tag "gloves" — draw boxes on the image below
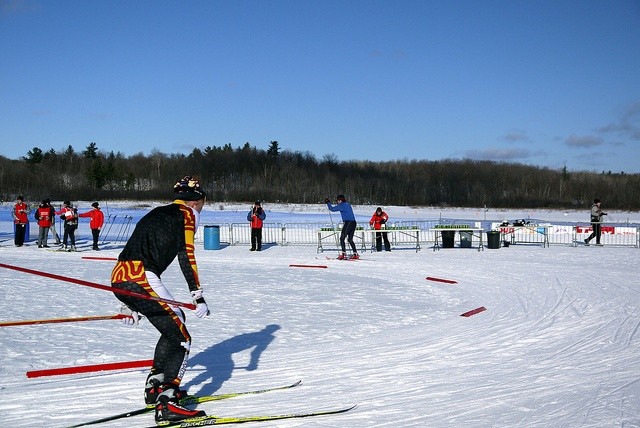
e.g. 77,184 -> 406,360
190,290 -> 208,319
120,303 -> 139,326
325,198 -> 330,203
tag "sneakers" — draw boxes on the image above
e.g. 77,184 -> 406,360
338,255 -> 347,260
70,245 -> 76,250
59,244 -> 66,250
155,383 -> 206,426
350,254 -> 359,259
144,369 -> 187,409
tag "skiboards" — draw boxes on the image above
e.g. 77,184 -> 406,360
46,249 -> 84,252
56,380 -> 356,427
317,256 -> 368,261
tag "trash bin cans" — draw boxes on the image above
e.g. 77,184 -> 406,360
487,231 -> 500,248
14,220 -> 30,242
441,231 -> 455,248
204,225 -> 220,250
459,231 -> 473,248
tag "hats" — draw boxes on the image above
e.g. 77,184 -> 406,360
172,175 -> 206,201
594,199 -> 600,204
91,202 -> 99,209
337,194 -> 344,198
17,196 -> 23,201
255,202 -> 261,205
64,201 -> 70,205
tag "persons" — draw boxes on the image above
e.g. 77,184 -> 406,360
585,200 -> 607,247
11,195 -> 31,248
369,207 -> 392,252
247,199 -> 266,251
325,193 -> 360,260
109,174 -> 208,425
79,202 -> 105,251
34,198 -> 55,249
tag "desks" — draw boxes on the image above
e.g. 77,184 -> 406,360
497,223 -> 552,248
366,229 -> 421,253
429,228 -> 484,251
317,230 -> 366,253
